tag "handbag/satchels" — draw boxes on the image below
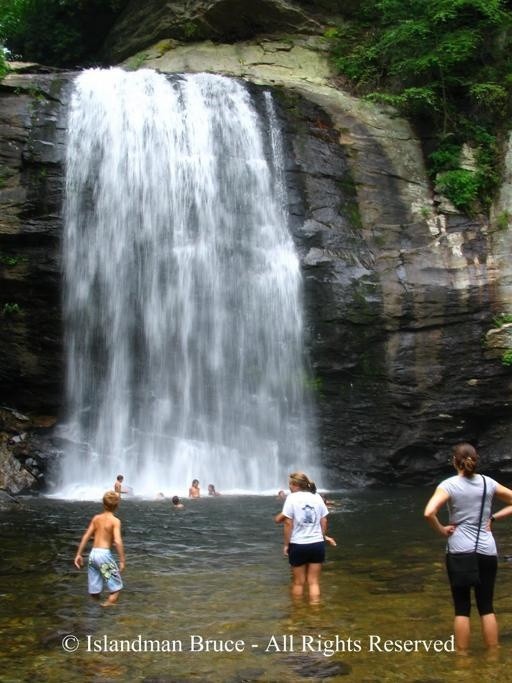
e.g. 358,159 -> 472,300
445,554 -> 476,574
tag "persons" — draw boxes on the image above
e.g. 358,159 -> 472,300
73,490 -> 126,604
274,472 -> 337,596
114,474 -> 128,499
423,442 -> 512,653
207,483 -> 221,497
171,495 -> 184,508
155,492 -> 164,500
188,479 -> 201,498
291,596 -> 320,604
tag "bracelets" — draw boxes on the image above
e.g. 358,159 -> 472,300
489,515 -> 495,522
